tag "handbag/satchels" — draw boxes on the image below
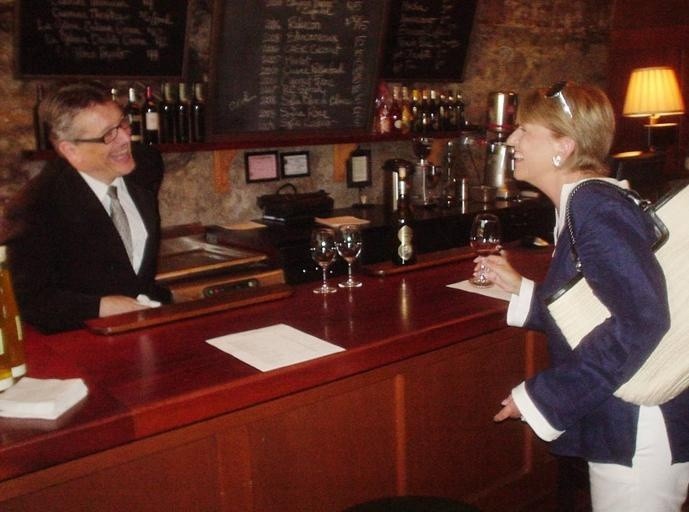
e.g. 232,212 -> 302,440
544,178 -> 689,407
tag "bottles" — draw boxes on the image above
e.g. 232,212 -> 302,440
420,86 -> 430,131
392,176 -> 417,266
410,88 -> 422,133
176,82 -> 190,143
375,95 -> 390,134
438,93 -> 448,131
429,88 -> 438,132
32,84 -> 52,150
159,81 -> 175,142
124,87 -> 143,144
190,81 -> 205,143
401,86 -> 410,132
142,86 -> 159,144
455,89 -> 465,130
389,86 -> 401,133
447,89 -> 457,131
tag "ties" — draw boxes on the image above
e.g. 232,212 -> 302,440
107,186 -> 134,269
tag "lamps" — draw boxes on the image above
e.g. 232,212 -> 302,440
622,67 -> 685,123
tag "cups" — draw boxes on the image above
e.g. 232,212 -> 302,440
337,224 -> 363,288
472,213 -> 502,286
311,228 -> 337,294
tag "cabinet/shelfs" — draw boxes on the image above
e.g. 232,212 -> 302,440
22,123 -> 487,163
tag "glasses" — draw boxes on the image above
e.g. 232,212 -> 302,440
56,113 -> 131,145
542,78 -> 573,119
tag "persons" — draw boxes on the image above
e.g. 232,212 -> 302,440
6,83 -> 173,334
472,80 -> 689,512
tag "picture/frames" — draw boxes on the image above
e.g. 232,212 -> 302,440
345,147 -> 373,188
280,152 -> 311,179
244,151 -> 280,184
